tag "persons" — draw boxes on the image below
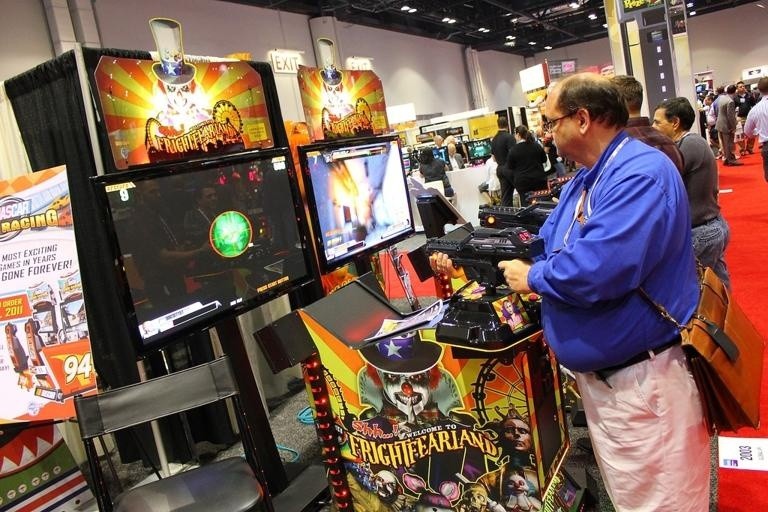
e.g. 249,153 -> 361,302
502,297 -> 527,331
506,125 -> 546,207
696,76 -> 768,182
428,71 -> 711,512
609,75 -> 684,176
650,97 -> 730,295
491,116 -> 529,208
187,183 -> 270,285
419,134 -> 464,206
476,417 -> 539,498
127,181 -> 238,307
529,125 -> 579,180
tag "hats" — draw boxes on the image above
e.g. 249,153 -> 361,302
359,329 -> 444,377
315,37 -> 343,85
145,16 -> 197,88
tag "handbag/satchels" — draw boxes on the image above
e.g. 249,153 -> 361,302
681,265 -> 768,433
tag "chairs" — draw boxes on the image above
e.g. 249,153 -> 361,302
73,354 -> 276,512
424,180 -> 457,208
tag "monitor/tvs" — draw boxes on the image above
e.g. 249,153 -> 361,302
401,154 -> 411,169
89,147 -> 320,351
465,139 -> 493,161
297,133 -> 416,274
432,146 -> 449,165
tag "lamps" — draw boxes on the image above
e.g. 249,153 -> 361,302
685,1 -> 697,16
401,5 -> 554,50
568,0 -> 608,29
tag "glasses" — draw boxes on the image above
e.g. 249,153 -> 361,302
543,112 -> 577,133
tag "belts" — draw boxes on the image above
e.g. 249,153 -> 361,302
595,336 -> 680,376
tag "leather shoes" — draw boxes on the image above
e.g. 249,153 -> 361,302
729,162 -> 743,165
